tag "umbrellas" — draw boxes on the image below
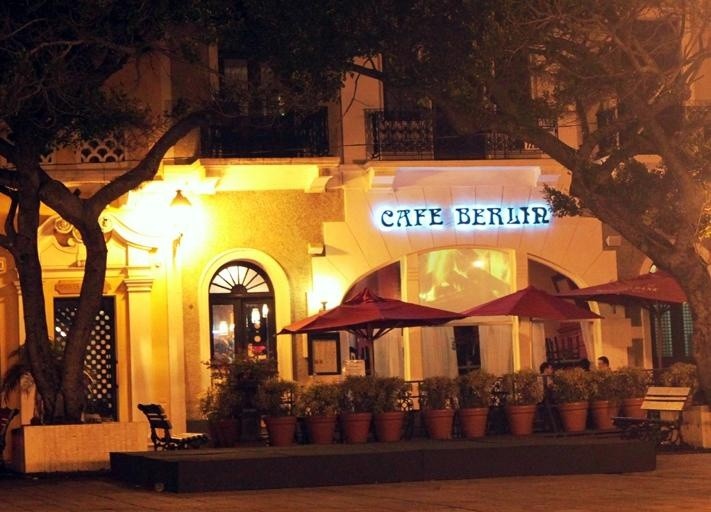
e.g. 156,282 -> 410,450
554,270 -> 689,369
273,286 -> 471,377
460,282 -> 606,369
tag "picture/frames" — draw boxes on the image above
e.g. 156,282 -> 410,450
307,333 -> 341,374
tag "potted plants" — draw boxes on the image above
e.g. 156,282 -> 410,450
201,348 -> 277,447
271,365 -> 646,445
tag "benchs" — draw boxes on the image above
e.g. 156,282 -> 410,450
611,387 -> 691,450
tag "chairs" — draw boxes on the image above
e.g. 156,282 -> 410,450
137,404 -> 209,451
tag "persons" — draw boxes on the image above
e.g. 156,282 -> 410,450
599,356 -> 613,374
539,363 -> 553,375
579,358 -> 592,372
